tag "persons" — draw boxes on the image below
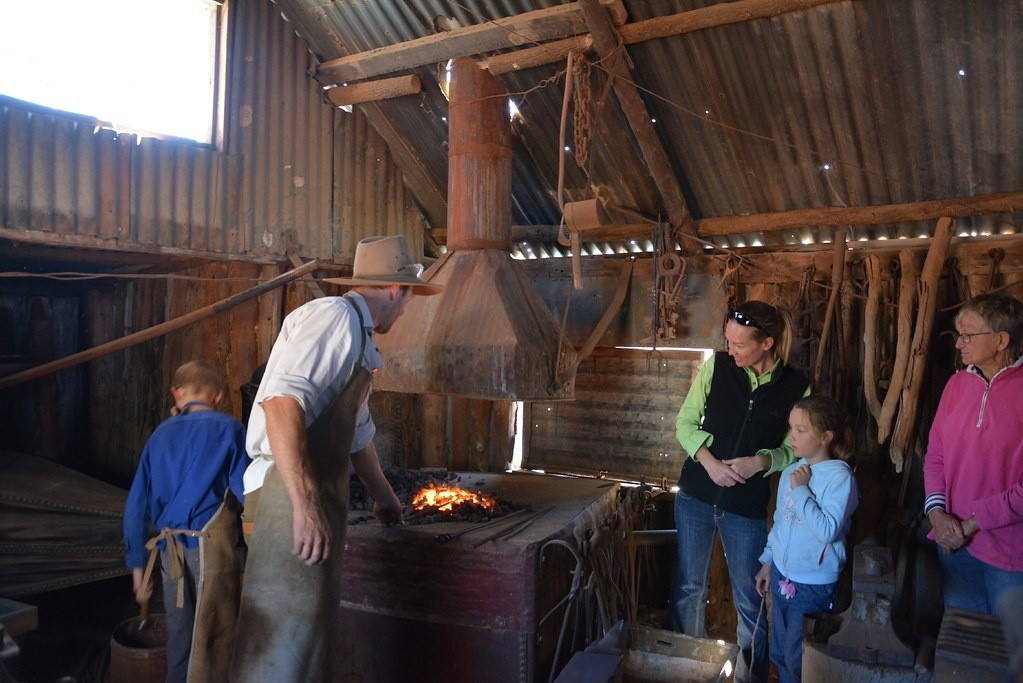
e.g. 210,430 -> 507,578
922,291 -> 1023,682
227,234 -> 451,683
120,359 -> 249,683
674,300 -> 813,683
754,394 -> 862,683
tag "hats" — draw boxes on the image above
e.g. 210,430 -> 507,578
323,234 -> 445,296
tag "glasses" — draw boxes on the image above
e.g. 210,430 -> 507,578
951,329 -> 993,344
727,310 -> 770,337
